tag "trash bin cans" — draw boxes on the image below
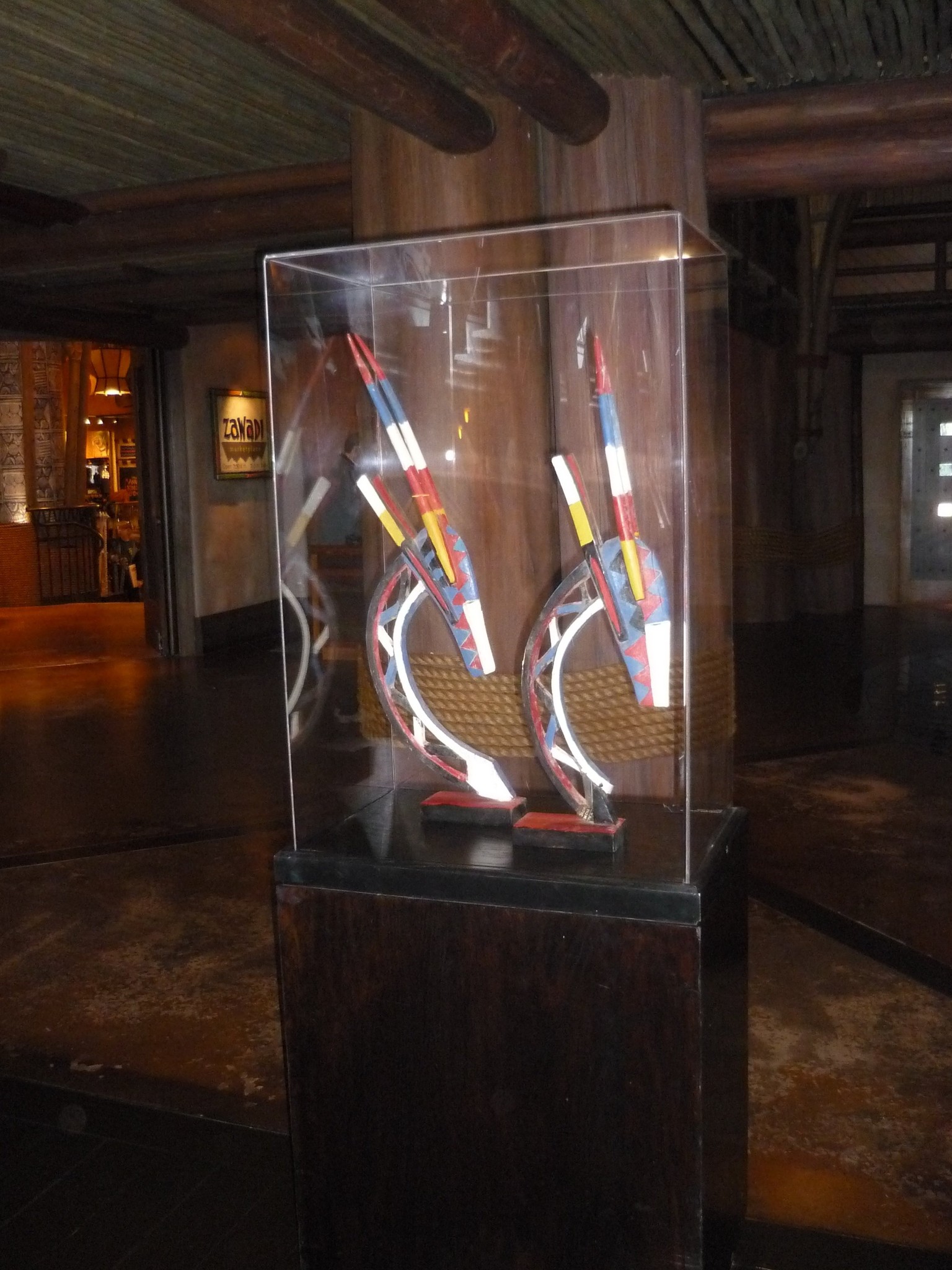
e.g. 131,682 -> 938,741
26,502 -> 110,604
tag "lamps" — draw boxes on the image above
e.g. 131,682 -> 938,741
96,417 -> 103,425
90,342 -> 131,396
